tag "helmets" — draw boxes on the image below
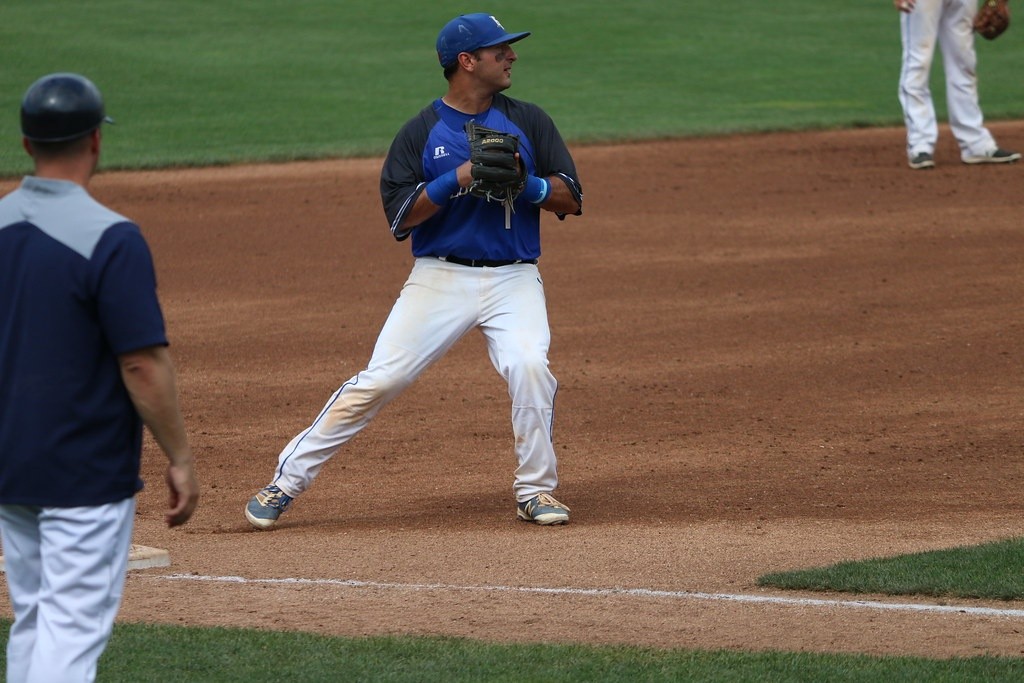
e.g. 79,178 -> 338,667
21,73 -> 115,141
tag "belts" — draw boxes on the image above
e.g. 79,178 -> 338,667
426,252 -> 536,267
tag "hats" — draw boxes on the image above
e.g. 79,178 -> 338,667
436,13 -> 531,68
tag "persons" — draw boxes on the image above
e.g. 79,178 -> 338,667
894,0 -> 1022,170
245,13 -> 584,532
0,73 -> 200,683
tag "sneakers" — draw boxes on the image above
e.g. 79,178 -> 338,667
516,493 -> 571,525
961,148 -> 1022,164
245,481 -> 294,531
908,152 -> 934,168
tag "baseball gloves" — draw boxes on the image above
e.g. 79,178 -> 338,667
461,118 -> 530,199
973,0 -> 1010,41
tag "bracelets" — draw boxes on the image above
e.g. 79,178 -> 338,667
425,168 -> 461,207
517,174 -> 551,208
